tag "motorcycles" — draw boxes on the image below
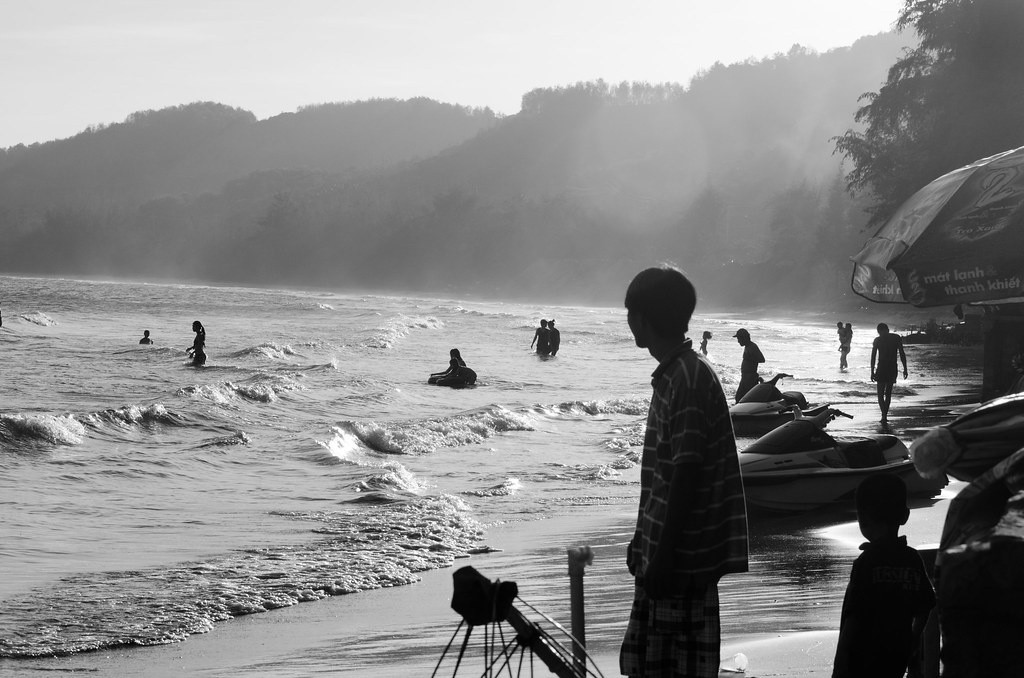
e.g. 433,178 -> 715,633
731,373 -> 811,428
740,402 -> 921,511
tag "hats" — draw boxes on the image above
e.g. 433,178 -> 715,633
732,328 -> 749,338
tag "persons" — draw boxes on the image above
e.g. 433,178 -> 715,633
832,474 -> 938,678
430,349 -> 477,385
620,267 -> 749,678
186,321 -> 206,364
870,323 -> 909,422
531,319 -> 560,356
837,321 -> 853,368
732,328 -> 765,403
140,330 -> 153,344
700,331 -> 712,356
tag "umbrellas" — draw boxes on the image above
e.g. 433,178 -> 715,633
849,146 -> 1024,308
